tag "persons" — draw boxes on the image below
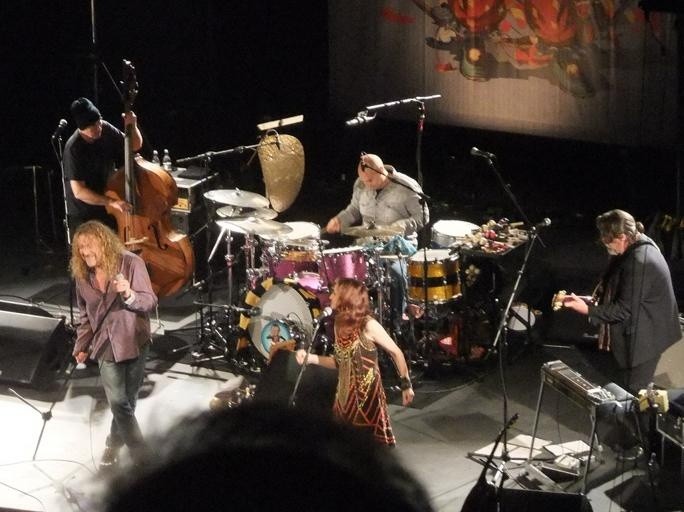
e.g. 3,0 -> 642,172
563,208 -> 683,463
264,323 -> 286,343
295,277 -> 416,449
326,154 -> 430,337
60,97 -> 144,246
66,218 -> 160,479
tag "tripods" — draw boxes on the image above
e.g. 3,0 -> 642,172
57,139 -> 84,329
408,199 -> 463,395
167,200 -> 287,376
366,251 -> 408,393
9,293 -> 119,460
504,239 -> 577,367
467,235 -> 555,484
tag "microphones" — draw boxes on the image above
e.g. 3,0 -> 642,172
471,147 -> 496,159
360,152 -> 366,172
275,136 -> 282,151
529,217 -> 552,232
117,273 -> 125,306
52,118 -> 67,139
310,307 -> 332,323
344,111 -> 377,126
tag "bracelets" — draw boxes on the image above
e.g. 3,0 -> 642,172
400,375 -> 411,390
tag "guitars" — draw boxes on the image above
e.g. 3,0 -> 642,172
551,281 -> 605,327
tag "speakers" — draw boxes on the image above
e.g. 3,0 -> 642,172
461,485 -> 594,512
0,310 -> 65,392
0,298 -> 53,318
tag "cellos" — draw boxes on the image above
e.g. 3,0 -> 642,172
104,58 -> 196,299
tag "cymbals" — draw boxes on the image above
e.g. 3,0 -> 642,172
203,190 -> 270,208
215,206 -> 293,236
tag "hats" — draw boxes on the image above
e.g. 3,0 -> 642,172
69,97 -> 102,131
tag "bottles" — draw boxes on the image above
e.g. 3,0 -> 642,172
162,148 -> 171,173
151,150 -> 160,166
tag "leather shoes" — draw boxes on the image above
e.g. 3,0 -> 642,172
620,444 -> 653,460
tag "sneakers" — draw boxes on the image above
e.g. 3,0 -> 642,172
99,437 -> 119,471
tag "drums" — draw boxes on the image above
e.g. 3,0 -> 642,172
406,248 -> 463,306
246,270 -> 337,361
315,246 -> 369,294
260,221 -> 330,284
428,219 -> 481,249
